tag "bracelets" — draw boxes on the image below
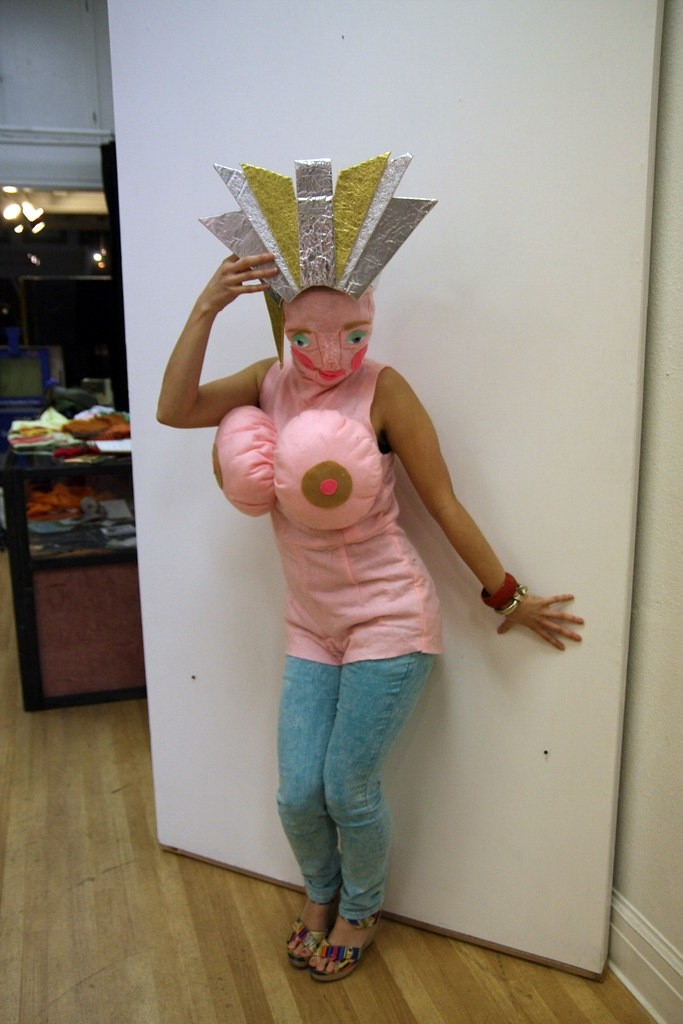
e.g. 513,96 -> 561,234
480,573 -> 527,614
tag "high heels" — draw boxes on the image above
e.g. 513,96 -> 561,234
308,909 -> 381,981
287,896 -> 339,968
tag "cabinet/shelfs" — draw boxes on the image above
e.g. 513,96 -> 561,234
0,448 -> 146,714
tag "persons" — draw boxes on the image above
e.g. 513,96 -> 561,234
157,153 -> 584,982
